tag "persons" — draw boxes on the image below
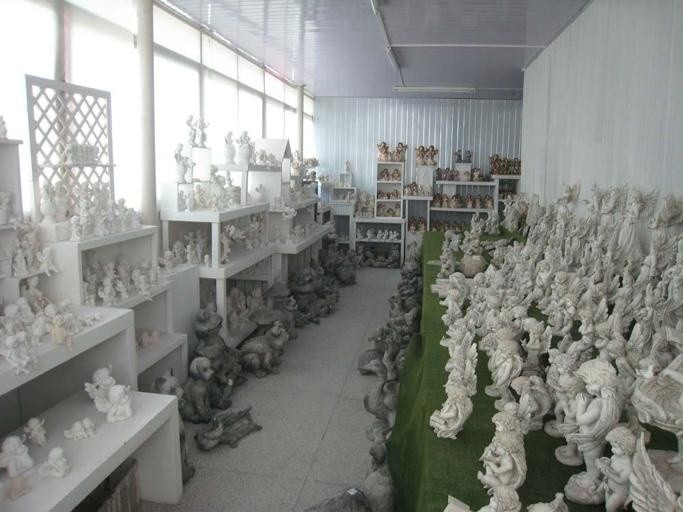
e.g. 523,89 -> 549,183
0,115 -> 683,512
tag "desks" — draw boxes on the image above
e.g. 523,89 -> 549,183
388,227 -> 678,512
